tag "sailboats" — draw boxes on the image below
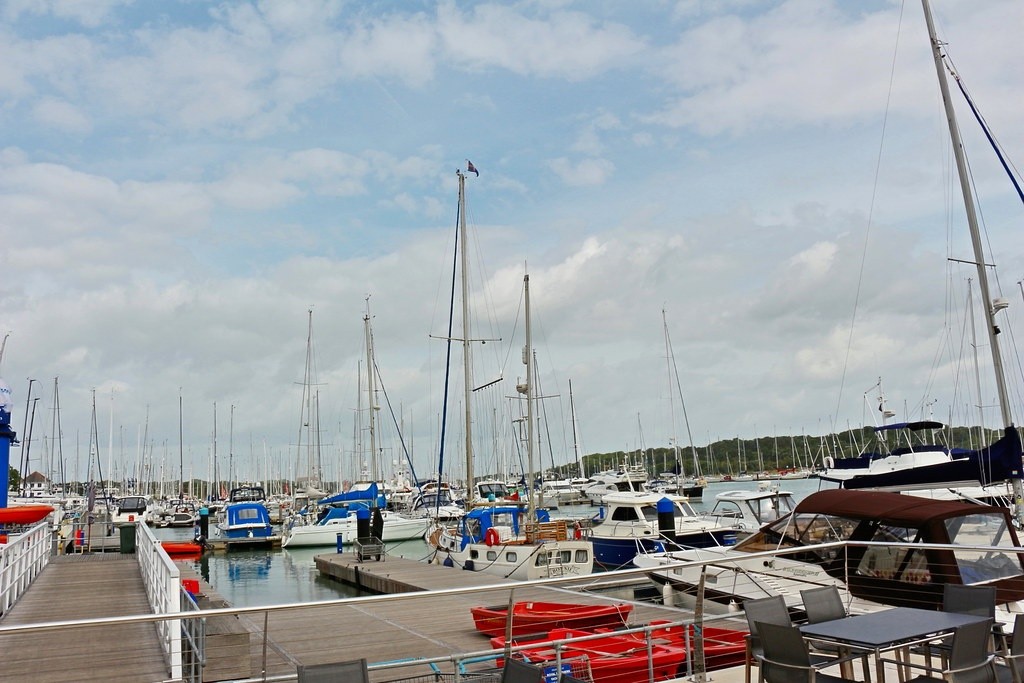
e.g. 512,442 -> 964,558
1,0 -> 1023,639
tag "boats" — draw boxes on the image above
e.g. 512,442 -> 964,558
488,626 -> 689,683
592,618 -> 757,669
470,600 -> 635,639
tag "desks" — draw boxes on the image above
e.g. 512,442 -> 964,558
800,605 -> 991,683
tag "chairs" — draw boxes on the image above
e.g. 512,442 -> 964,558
743,584 -> 1024,683
